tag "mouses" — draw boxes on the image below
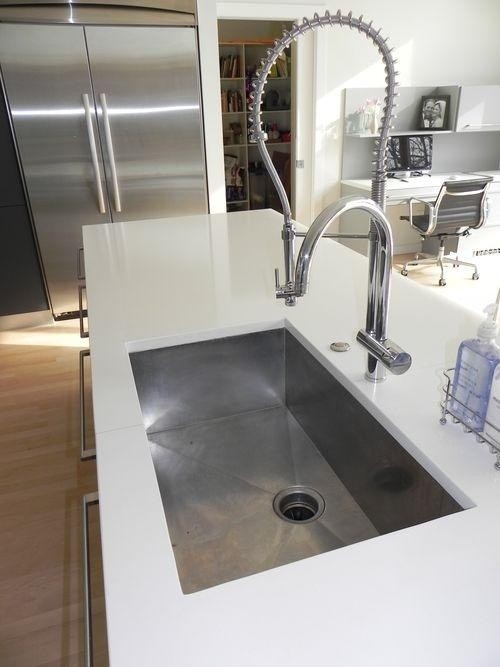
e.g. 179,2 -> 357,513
449,174 -> 460,181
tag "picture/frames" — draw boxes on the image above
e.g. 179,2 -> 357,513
417,93 -> 451,129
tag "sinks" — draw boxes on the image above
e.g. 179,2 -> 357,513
122,318 -> 476,594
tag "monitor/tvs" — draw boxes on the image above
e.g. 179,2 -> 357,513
384,134 -> 434,182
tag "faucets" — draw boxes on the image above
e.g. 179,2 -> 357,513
294,194 -> 413,383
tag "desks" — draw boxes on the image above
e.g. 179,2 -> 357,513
336,167 -> 500,255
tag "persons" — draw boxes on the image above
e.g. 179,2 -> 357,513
429,100 -> 446,128
422,98 -> 436,128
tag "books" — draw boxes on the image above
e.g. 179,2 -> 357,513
218,50 -> 291,213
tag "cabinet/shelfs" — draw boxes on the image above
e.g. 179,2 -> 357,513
218,39 -> 294,216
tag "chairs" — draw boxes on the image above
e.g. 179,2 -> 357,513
398,176 -> 492,286
